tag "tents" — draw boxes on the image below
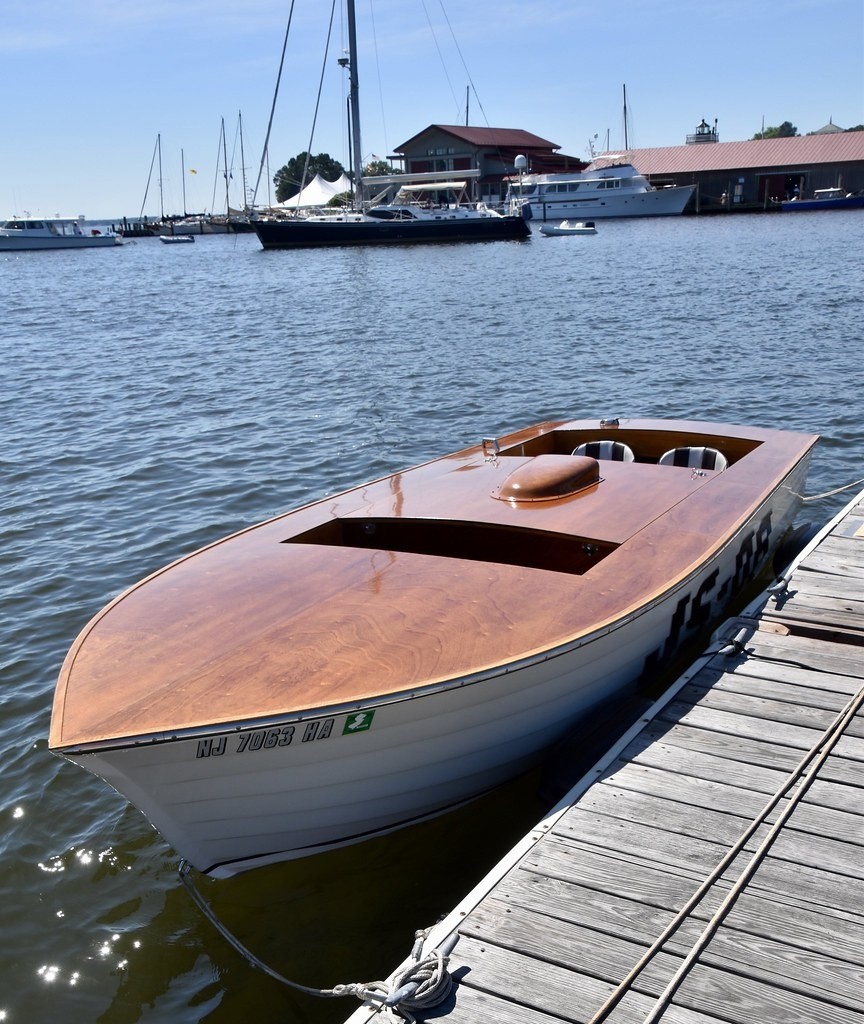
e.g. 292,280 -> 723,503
270,171 -> 358,219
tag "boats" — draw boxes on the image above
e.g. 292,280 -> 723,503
502,154 -> 698,217
159,234 -> 195,244
44,411 -> 823,882
781,187 -> 864,212
0,219 -> 125,252
540,221 -> 598,236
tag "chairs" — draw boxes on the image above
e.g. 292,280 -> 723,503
571,440 -> 635,462
657,446 -> 728,471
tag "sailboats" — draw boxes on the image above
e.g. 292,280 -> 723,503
110,110 -> 271,239
246,0 -> 537,249
